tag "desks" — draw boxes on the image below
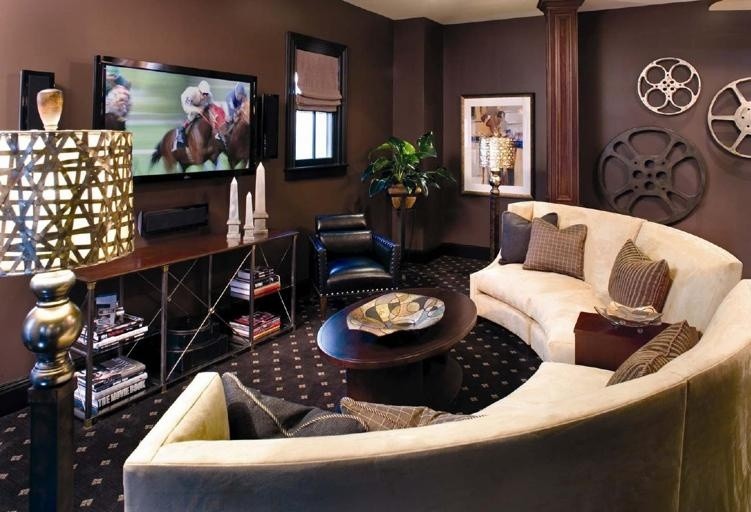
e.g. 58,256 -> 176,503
71,229 -> 299,428
574,312 -> 673,372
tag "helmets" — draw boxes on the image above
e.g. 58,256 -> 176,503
235,84 -> 246,97
198,80 -> 210,93
108,68 -> 119,75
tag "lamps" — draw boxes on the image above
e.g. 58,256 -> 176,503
479,136 -> 515,263
0,88 -> 136,511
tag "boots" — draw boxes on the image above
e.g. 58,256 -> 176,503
177,120 -> 189,144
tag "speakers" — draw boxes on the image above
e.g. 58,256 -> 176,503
259,93 -> 280,160
18,68 -> 55,130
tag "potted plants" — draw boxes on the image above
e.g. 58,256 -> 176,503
361,129 -> 456,210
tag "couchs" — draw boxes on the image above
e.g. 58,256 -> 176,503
469,199 -> 744,363
306,212 -> 401,325
122,278 -> 751,512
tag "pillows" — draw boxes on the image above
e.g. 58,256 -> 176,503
523,217 -> 588,281
221,371 -> 368,442
337,396 -> 486,430
608,238 -> 671,312
498,211 -> 559,265
606,319 -> 699,386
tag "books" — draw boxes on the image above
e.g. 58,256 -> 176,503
229,266 -> 281,296
73,355 -> 149,415
229,310 -> 281,340
76,294 -> 149,351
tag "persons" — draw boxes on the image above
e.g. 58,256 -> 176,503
106,66 -> 132,95
225,82 -> 247,122
176,79 -> 214,145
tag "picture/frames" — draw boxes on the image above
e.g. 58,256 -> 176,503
459,92 -> 535,199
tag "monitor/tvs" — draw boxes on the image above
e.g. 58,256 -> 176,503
92,54 -> 259,190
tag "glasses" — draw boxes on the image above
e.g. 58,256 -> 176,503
199,89 -> 209,96
110,74 -> 117,78
